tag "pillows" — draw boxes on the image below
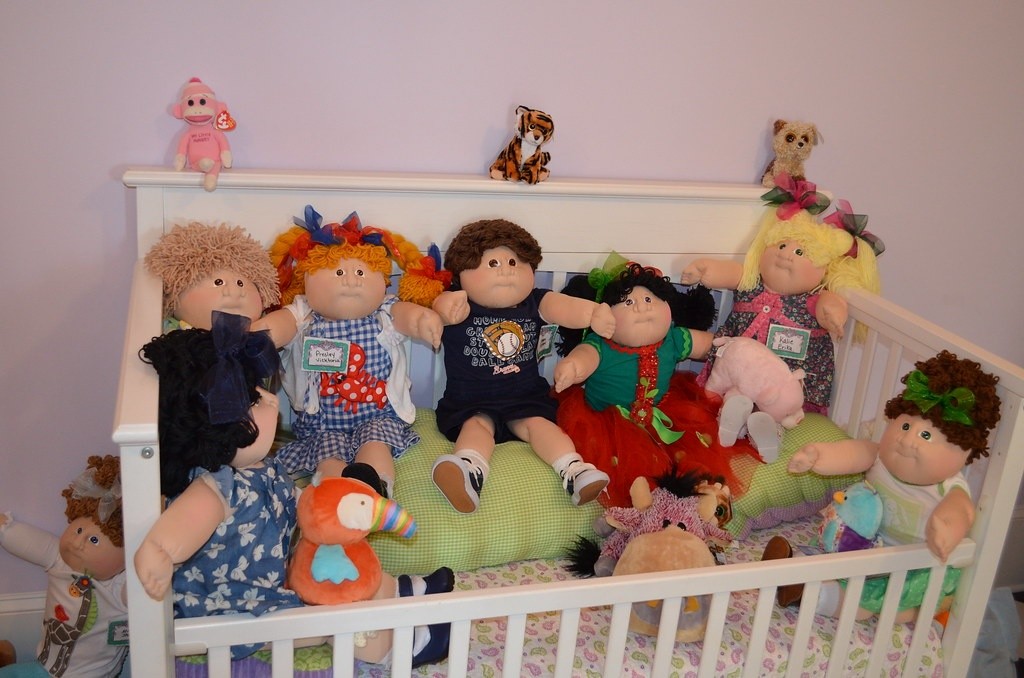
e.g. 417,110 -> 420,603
368,409 -> 608,577
722,414 -> 865,541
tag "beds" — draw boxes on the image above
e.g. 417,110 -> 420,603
112,166 -> 1024,678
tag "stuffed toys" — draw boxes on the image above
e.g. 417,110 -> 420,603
0,77 -> 1003,678
432,219 -> 616,514
245,205 -> 453,499
